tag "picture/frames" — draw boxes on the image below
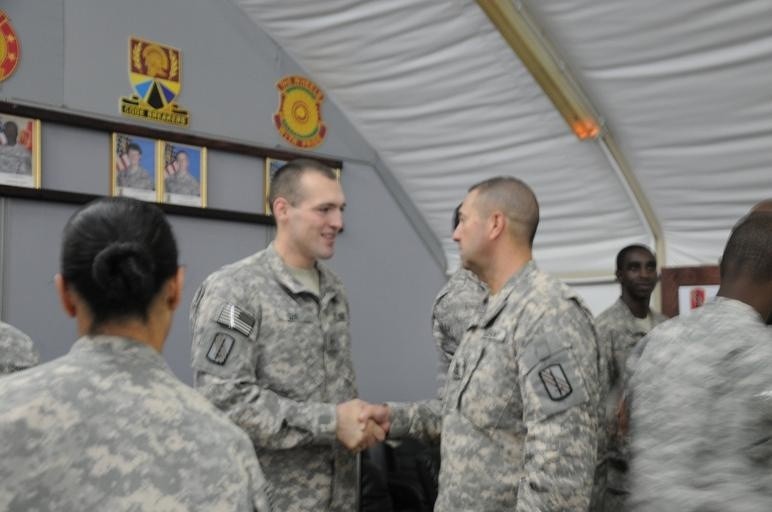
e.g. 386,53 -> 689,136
110,130 -> 161,204
0,112 -> 43,191
158,138 -> 209,211
266,155 -> 289,218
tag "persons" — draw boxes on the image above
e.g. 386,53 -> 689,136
621,196 -> 772,512
193,155 -> 385,511
359,175 -> 607,512
117,143 -> 153,190
0,197 -> 268,510
167,151 -> 200,197
0,123 -> 32,175
429,201 -> 493,393
592,244 -> 670,510
